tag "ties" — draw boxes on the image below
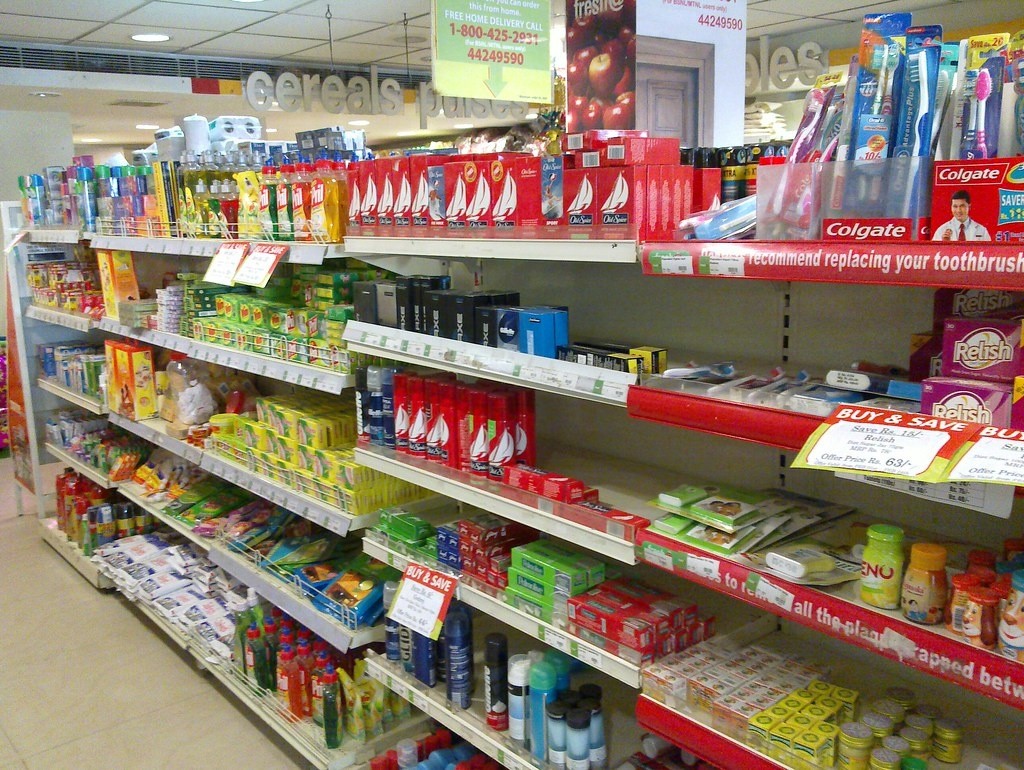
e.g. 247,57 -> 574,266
959,224 -> 966,241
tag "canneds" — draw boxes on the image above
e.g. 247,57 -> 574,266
837,686 -> 962,770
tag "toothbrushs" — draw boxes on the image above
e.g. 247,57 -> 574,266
678,11 -> 1024,242
649,360 -> 823,406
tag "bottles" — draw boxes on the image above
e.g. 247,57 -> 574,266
261,160 -> 350,243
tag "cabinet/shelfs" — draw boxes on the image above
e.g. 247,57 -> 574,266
343,224 -> 1024,770
1,199 -> 163,592
90,216 -> 461,770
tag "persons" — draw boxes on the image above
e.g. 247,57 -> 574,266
696,501 -> 742,547
932,191 -> 992,241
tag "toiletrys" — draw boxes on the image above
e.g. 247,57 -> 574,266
353,276 -> 569,361
355,364 -> 537,480
383,582 -> 606,770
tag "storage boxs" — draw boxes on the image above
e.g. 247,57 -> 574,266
348,129 -> 721,243
35,339 -> 106,400
814,287 -> 1023,435
372,503 -> 859,769
95,248 -> 159,329
215,393 -> 441,515
238,126 -> 368,164
46,411 -> 151,481
26,259 -> 105,313
103,339 -> 157,421
95,162 -> 184,236
177,257 -> 409,373
354,270 -> 667,383
756,156 -> 1024,242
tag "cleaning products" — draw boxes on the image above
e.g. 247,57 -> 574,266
17,153 -> 174,236
232,586 -> 424,750
55,466 -> 163,556
176,152 -> 349,243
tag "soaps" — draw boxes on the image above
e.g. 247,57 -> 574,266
215,389 -> 435,515
176,257 -> 396,374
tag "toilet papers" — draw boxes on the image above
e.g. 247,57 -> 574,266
102,151 -> 129,166
130,116 -> 298,167
